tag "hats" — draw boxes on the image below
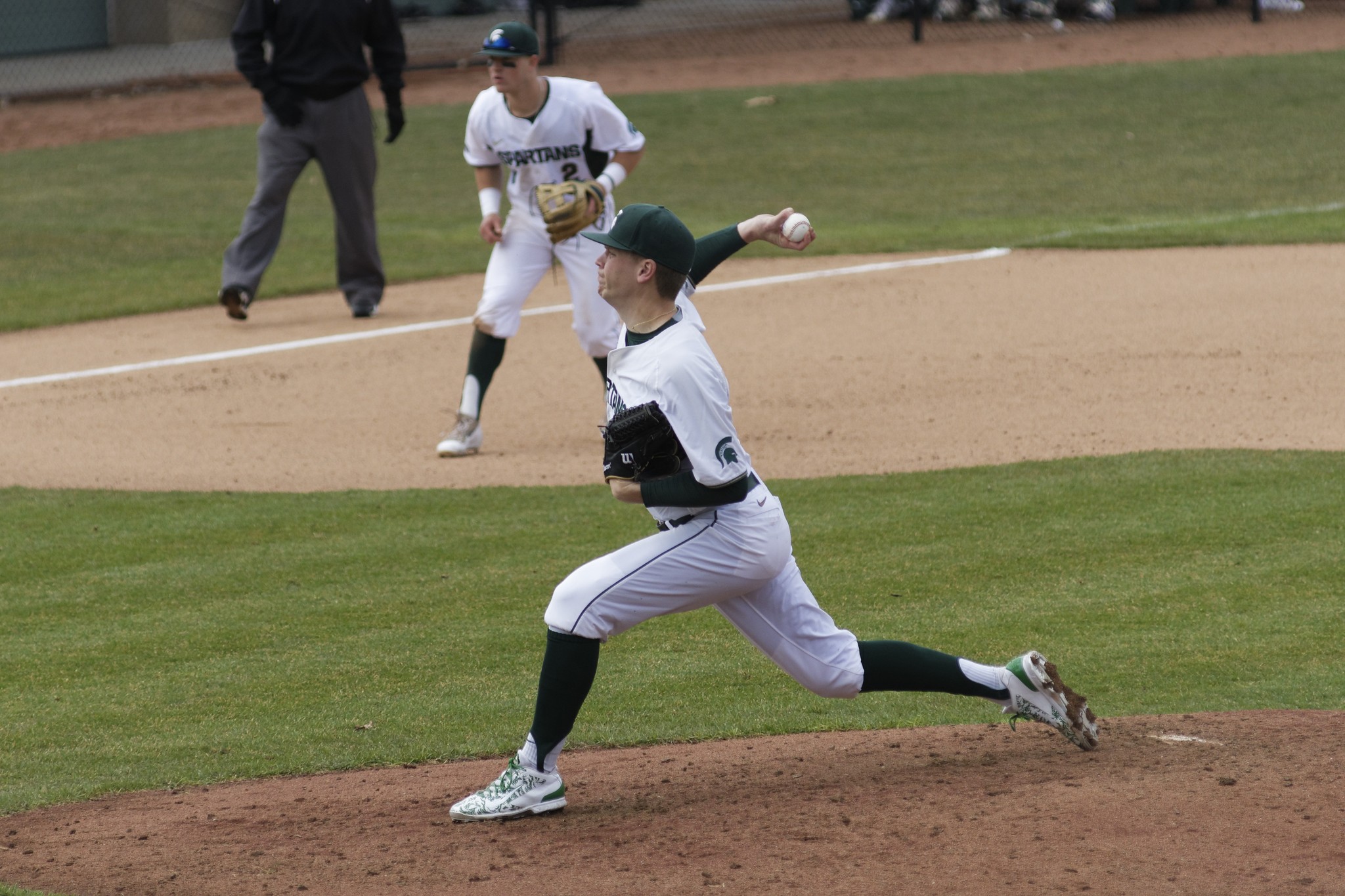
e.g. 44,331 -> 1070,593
580,202 -> 698,274
474,21 -> 540,59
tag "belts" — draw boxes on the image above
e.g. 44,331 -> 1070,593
656,472 -> 758,531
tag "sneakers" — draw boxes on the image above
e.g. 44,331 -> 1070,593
434,411 -> 481,456
998,649 -> 1098,751
449,748 -> 569,822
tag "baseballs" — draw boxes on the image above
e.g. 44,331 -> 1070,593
782,211 -> 812,244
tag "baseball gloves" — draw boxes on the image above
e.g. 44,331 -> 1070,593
602,402 -> 681,482
536,179 -> 606,245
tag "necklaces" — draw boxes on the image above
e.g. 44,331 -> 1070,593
626,309 -> 672,328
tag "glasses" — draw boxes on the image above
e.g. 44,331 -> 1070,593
483,37 -> 534,55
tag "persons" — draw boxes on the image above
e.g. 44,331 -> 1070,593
860,0 -> 1120,33
217,0 -> 411,320
437,18 -> 646,459
526,0 -> 558,66
447,205 -> 1101,827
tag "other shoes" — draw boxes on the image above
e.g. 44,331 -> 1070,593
352,302 -> 372,317
218,284 -> 247,321
